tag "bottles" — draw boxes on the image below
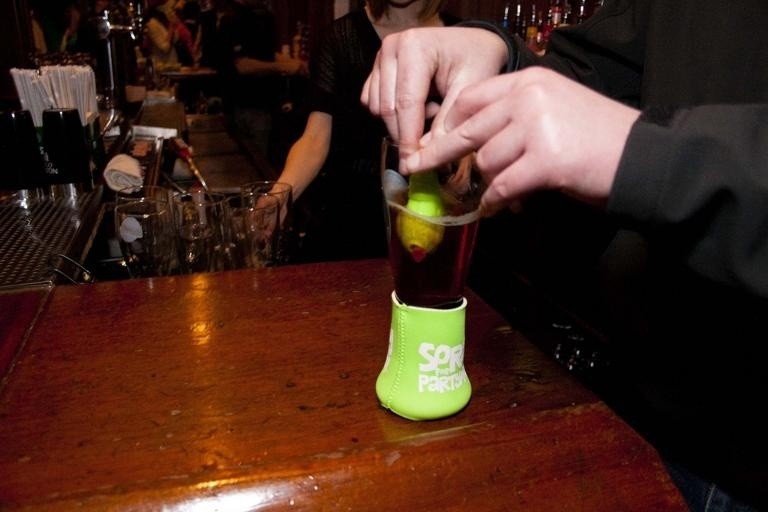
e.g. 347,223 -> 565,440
501,2 -> 591,55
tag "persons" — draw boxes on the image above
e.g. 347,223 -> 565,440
360,0 -> 767,300
250,0 -> 465,263
146,0 -> 311,114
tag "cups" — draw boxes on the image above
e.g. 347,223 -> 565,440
114,181 -> 294,276
375,132 -> 475,421
